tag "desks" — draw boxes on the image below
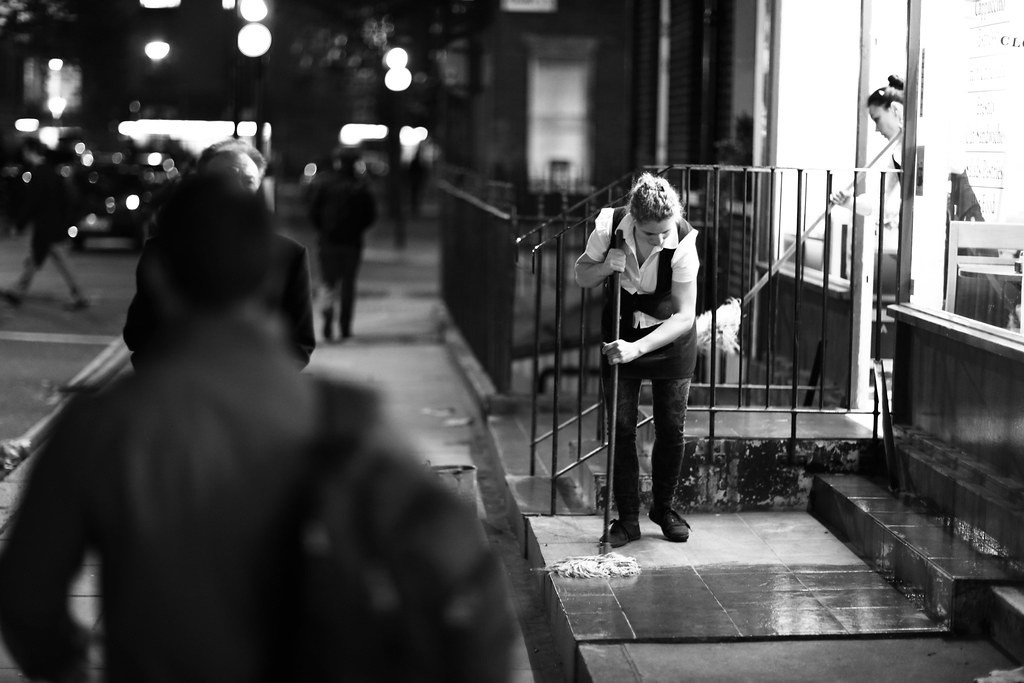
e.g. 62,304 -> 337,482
959,263 -> 1023,329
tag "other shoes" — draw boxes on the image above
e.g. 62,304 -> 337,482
339,325 -> 352,340
0,289 -> 20,306
68,297 -> 89,312
324,308 -> 335,341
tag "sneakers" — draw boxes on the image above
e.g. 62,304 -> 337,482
599,519 -> 641,546
649,506 -> 691,542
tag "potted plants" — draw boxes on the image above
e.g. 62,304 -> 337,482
715,109 -> 753,202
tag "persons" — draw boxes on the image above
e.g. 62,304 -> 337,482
573,171 -> 699,548
305,143 -> 434,341
1,142 -> 90,311
829,73 -> 999,328
199,141 -> 267,192
0,168 -> 518,683
